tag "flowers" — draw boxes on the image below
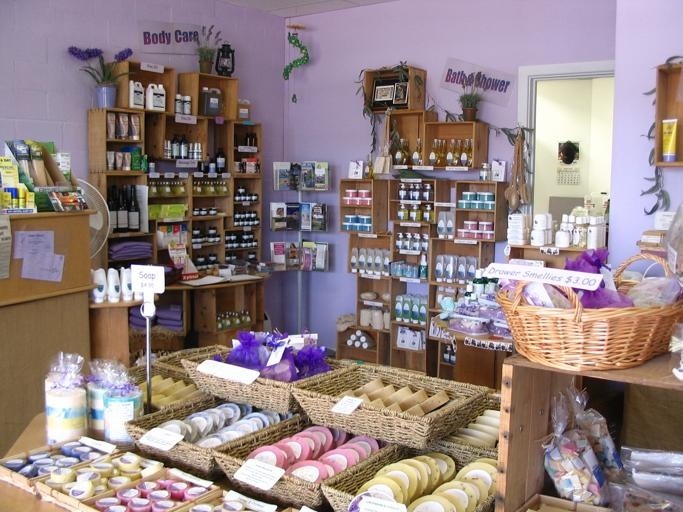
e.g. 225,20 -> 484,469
67,45 -> 133,84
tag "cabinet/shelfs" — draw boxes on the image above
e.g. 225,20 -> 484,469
438,242 -> 607,390
86,61 -> 264,366
336,65 -> 506,390
2,207 -> 97,459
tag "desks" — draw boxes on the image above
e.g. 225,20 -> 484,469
0,412 -> 67,511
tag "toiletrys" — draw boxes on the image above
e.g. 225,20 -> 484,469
106,81 -> 607,350
90,266 -> 143,302
341,137 -> 608,349
106,82 -> 261,350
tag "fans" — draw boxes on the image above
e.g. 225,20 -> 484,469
73,178 -> 112,259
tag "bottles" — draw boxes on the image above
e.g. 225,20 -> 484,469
359,306 -> 390,331
174,94 -> 192,114
464,270 -> 498,301
4,186 -> 25,210
234,160 -> 260,173
107,183 -> 139,236
392,256 -> 427,279
144,154 -> 155,173
555,192 -> 608,250
148,181 -> 228,197
121,147 -> 140,170
163,134 -> 226,174
394,137 -> 473,165
233,133 -> 256,148
216,310 -> 251,331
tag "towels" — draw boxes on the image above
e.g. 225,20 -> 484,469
108,240 -> 153,262
127,303 -> 182,336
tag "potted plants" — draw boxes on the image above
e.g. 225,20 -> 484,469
197,47 -> 214,74
455,93 -> 483,122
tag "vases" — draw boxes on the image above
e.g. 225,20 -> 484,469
95,84 -> 117,109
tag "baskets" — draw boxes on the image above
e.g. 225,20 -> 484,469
409,381 -> 499,468
179,340 -> 358,414
123,394 -> 301,481
156,341 -> 241,375
493,253 -> 683,374
120,362 -> 206,415
292,359 -> 491,449
319,436 -> 498,512
209,408 -> 393,511
